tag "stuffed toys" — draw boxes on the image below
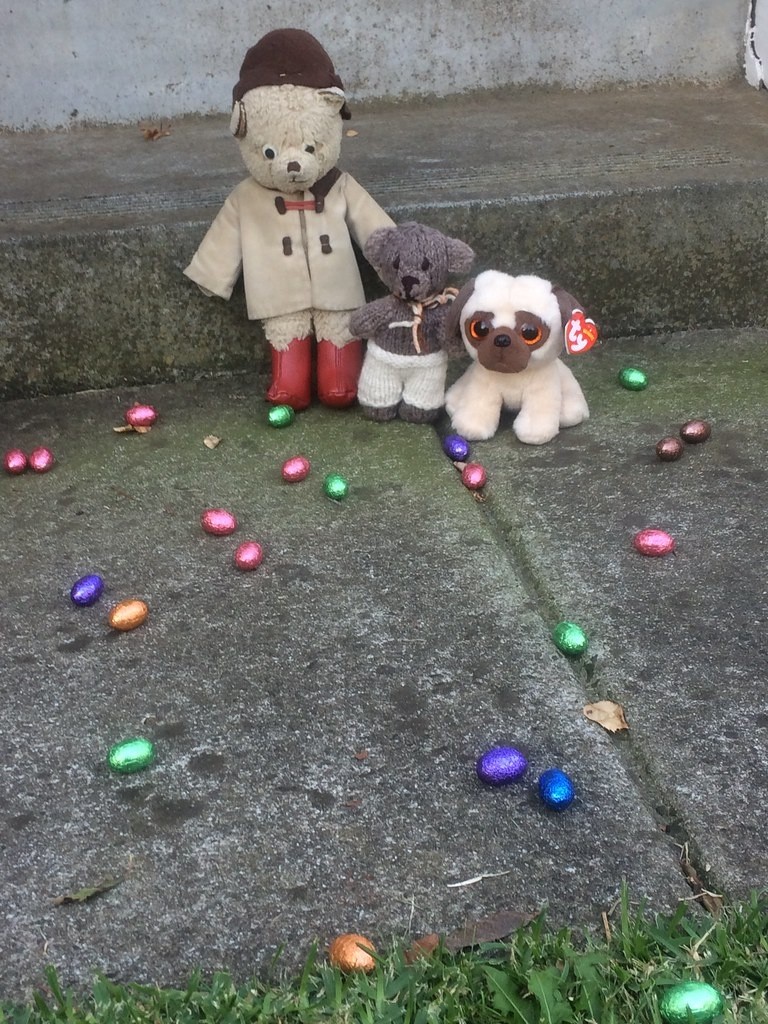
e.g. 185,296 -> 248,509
349,223 -> 477,424
181,26 -> 401,412
441,268 -> 599,447
